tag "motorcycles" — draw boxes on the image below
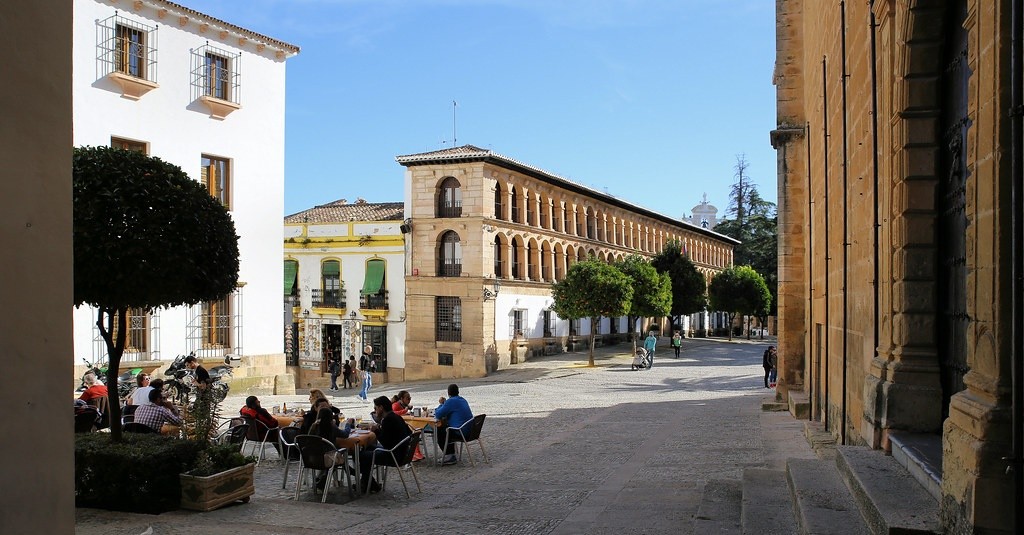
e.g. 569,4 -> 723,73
75,358 -> 143,398
163,351 -> 241,404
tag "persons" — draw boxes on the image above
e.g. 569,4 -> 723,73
672,331 -> 682,359
127,373 -> 192,432
435,384 -> 473,464
351,396 -> 409,493
301,389 -> 361,492
78,370 -> 108,402
644,331 -> 656,368
391,391 -> 425,462
184,356 -> 212,423
327,345 -> 375,404
240,396 -> 287,460
762,346 -> 777,389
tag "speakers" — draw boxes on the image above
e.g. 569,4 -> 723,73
400,224 -> 410,234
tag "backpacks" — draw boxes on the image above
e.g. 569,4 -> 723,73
358,355 -> 366,371
333,363 -> 341,376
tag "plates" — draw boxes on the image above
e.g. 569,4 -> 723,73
358,430 -> 371,433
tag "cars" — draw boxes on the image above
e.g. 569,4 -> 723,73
751,327 -> 769,336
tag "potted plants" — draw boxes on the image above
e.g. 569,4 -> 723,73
179,376 -> 259,510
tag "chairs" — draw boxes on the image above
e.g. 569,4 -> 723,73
75,397 -> 486,504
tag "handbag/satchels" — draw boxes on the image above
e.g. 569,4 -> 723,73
350,368 -> 356,374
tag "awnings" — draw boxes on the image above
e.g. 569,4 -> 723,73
323,262 -> 339,276
284,260 -> 297,295
361,260 -> 385,295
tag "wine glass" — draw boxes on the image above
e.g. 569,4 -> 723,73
356,415 -> 362,432
407,403 -> 413,416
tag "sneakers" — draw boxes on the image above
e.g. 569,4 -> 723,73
412,451 -> 425,461
438,455 -> 457,465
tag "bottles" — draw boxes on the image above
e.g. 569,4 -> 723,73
283,403 -> 287,413
414,408 -> 421,416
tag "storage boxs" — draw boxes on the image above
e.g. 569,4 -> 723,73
224,353 -> 242,367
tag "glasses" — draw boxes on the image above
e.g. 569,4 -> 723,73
143,377 -> 151,381
256,401 -> 260,403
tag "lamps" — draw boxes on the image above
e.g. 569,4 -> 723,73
350,310 -> 357,316
483,279 -> 500,299
482,224 -> 492,232
303,309 -> 309,314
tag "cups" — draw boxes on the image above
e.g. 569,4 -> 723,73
338,413 -> 345,424
273,406 -> 280,414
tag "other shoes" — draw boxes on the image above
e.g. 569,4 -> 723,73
765,386 -> 771,389
328,388 -> 339,392
315,477 -> 329,492
357,394 -> 363,401
289,457 -> 300,463
352,482 -> 382,493
770,383 -> 776,387
363,399 -> 371,404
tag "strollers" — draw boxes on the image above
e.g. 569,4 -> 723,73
632,347 -> 651,371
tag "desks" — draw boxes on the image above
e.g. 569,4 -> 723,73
269,413 -> 304,466
160,423 -> 182,436
335,430 -> 377,497
401,413 -> 444,467
